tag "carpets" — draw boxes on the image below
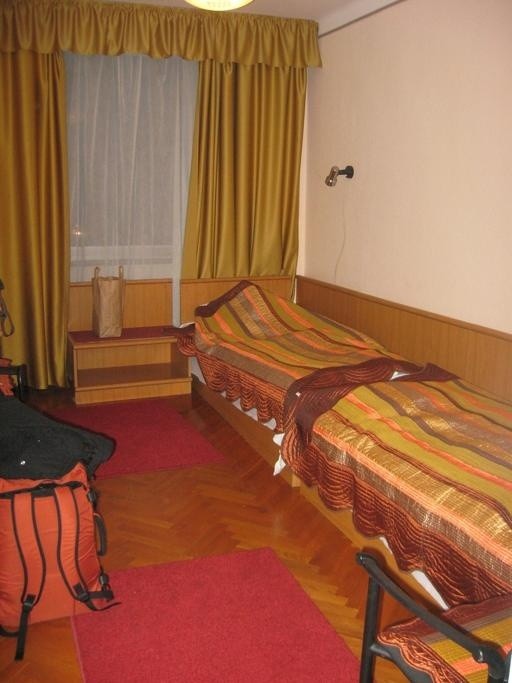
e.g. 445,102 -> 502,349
73,549 -> 363,683
45,397 -> 227,478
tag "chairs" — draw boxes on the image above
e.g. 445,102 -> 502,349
355,551 -> 512,683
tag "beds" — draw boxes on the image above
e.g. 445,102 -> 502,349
182,322 -> 423,486
301,376 -> 512,612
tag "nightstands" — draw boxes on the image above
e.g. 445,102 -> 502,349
66,326 -> 196,407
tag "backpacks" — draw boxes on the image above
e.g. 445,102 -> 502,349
0,461 -> 114,662
